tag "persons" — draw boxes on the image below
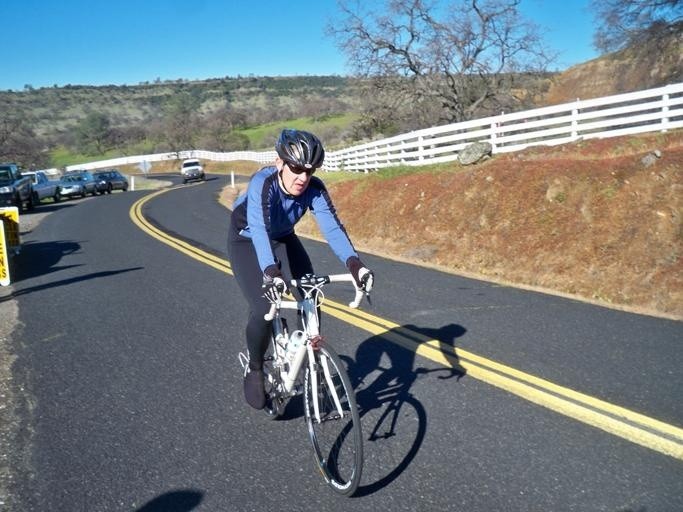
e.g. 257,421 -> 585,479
223,127 -> 375,427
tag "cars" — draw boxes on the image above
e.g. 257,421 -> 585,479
0,162 -> 128,210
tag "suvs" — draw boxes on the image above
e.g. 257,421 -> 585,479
181,159 -> 205,183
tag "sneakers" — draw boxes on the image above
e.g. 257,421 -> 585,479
243,370 -> 268,410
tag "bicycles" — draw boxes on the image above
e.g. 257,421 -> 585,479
248,269 -> 365,497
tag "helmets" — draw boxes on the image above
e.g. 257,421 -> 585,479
275,128 -> 325,168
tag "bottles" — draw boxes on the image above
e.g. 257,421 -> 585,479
285,329 -> 301,362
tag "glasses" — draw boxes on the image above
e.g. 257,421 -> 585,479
287,164 -> 316,174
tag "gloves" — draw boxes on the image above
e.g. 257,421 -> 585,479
347,255 -> 376,293
264,264 -> 302,302
